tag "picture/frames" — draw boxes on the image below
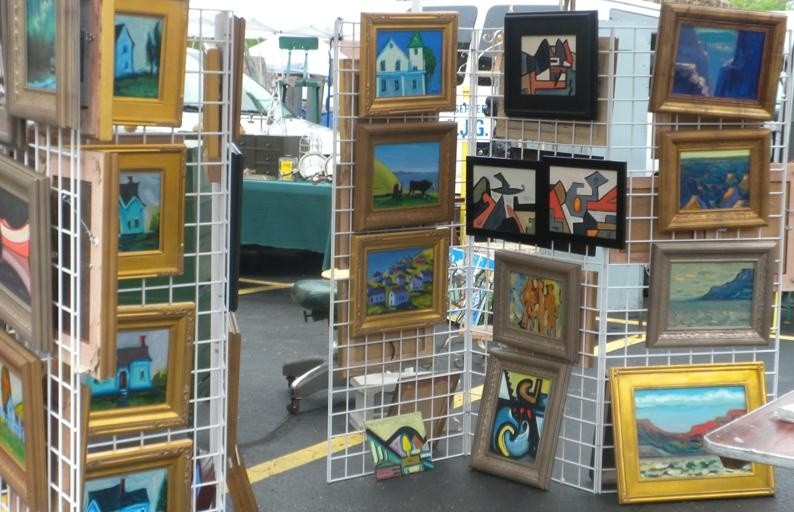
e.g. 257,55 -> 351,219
1,0 -> 195,512
538,153 -> 630,255
468,346 -> 571,493
607,360 -> 783,505
350,228 -> 456,338
463,153 -> 548,250
657,128 -> 776,233
353,120 -> 458,232
644,2 -> 786,125
361,410 -> 438,485
643,239 -> 782,350
358,9 -> 459,120
491,247 -> 583,365
500,9 -> 602,124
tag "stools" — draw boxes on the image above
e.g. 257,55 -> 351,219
281,277 -> 344,415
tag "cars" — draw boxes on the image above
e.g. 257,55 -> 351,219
26,45 -> 337,178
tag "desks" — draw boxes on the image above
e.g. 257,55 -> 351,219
704,383 -> 794,468
236,177 -> 334,278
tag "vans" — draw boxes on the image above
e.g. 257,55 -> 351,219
406,4 -> 794,189
